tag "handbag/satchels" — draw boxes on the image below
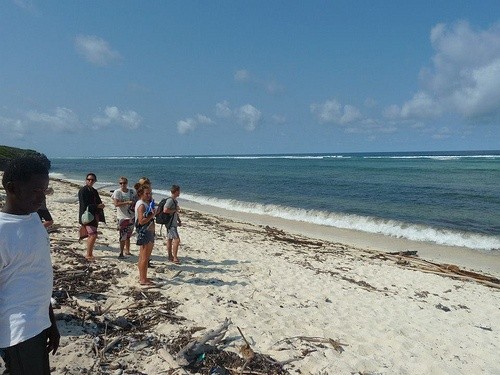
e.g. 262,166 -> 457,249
81,206 -> 93,224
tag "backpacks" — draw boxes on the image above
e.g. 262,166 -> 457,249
155,197 -> 176,233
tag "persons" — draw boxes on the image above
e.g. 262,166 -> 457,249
0,148 -> 60,375
78,174 -> 107,260
37,206 -> 54,227
112,176 -> 182,285
